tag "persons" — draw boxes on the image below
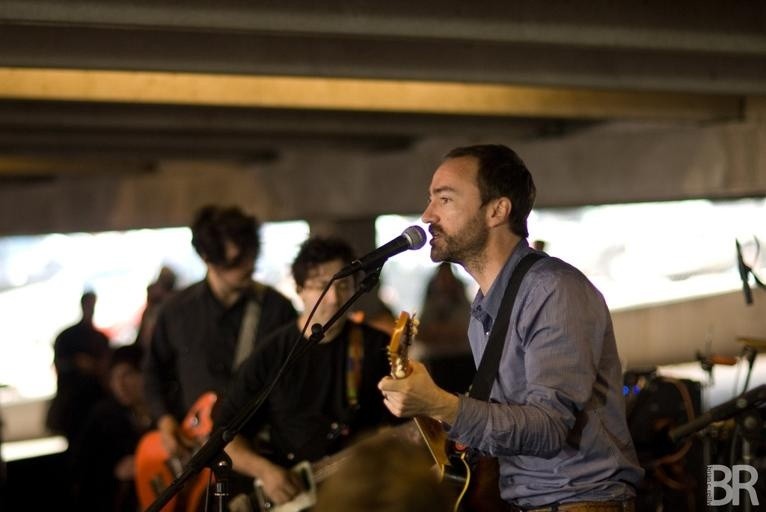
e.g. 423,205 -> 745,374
376,144 -> 644,512
45,201 -> 401,511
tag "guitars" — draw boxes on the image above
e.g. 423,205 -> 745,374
135,392 -> 220,512
219,422 -> 415,511
388,312 -> 499,512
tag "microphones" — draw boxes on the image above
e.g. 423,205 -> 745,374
336,225 -> 427,281
731,239 -> 755,305
